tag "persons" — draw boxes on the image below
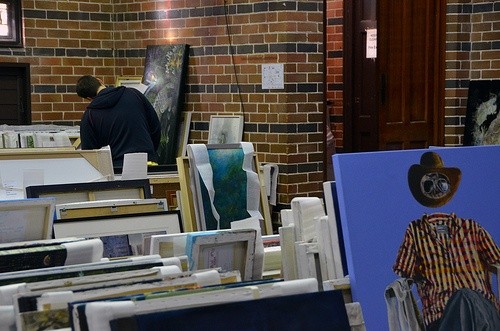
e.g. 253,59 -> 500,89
76,75 -> 162,167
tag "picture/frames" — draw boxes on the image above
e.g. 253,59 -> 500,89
0,44 -> 352,331
